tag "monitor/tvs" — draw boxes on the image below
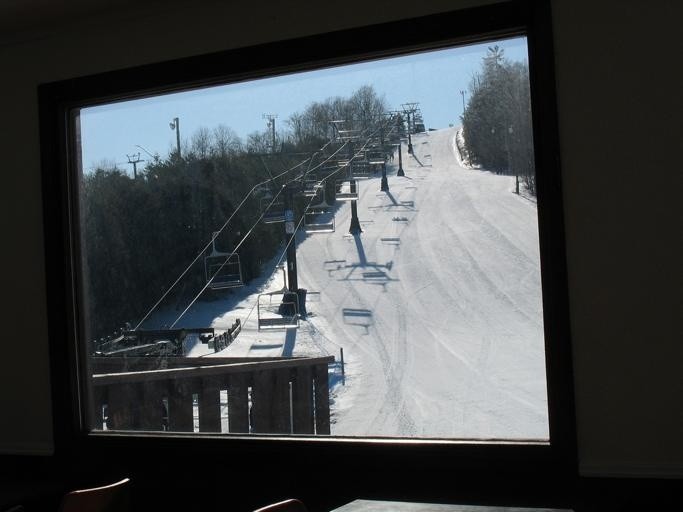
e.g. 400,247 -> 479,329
34,0 -> 580,492
